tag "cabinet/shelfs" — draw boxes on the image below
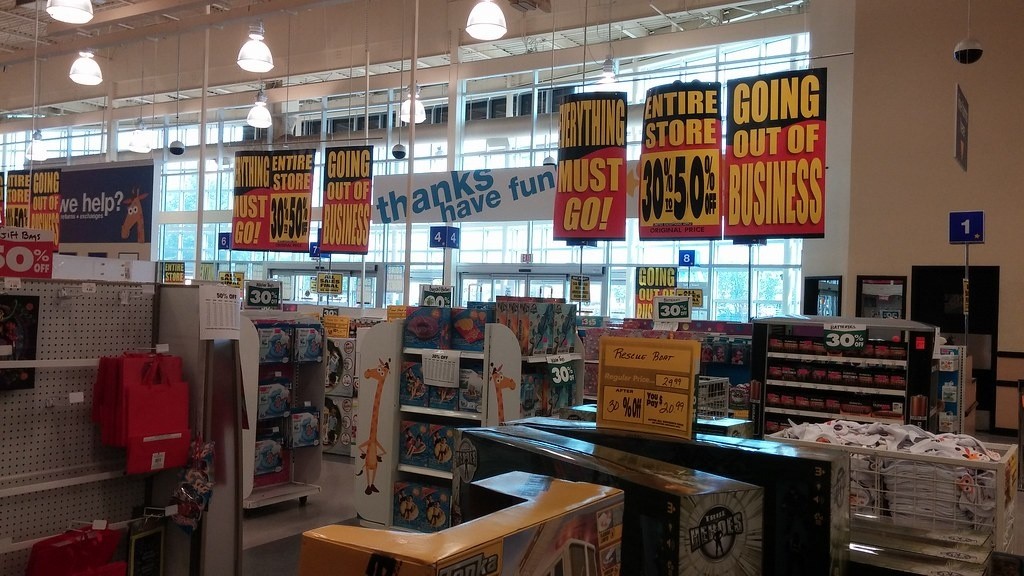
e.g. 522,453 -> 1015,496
742,316 -> 936,441
389,349 -> 588,535
236,308 -> 328,515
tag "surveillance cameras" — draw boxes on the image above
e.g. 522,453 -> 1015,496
954,38 -> 984,64
543,157 -> 556,165
392,144 -> 406,159
170,141 -> 185,155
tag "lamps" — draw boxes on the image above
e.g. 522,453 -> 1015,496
248,86 -> 273,128
44,0 -> 93,24
70,50 -> 103,85
399,79 -> 427,123
237,21 -> 274,72
465,2 -> 507,40
27,61 -> 48,161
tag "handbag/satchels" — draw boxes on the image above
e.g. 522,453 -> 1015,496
26,520 -> 127,576
89,352 -> 190,475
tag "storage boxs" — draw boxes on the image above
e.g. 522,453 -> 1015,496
297,415 -> 850,576
255,326 -> 324,475
395,296 -> 580,533
